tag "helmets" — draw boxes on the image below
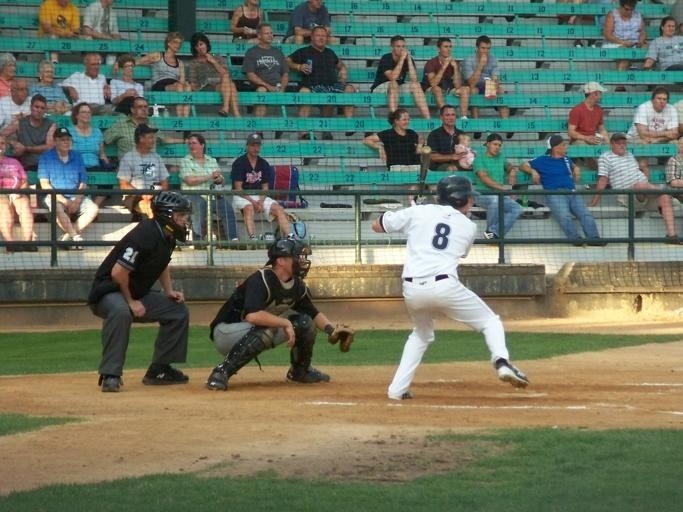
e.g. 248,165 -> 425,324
150,191 -> 191,242
267,237 -> 312,279
438,174 -> 483,201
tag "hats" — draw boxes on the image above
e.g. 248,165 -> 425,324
134,123 -> 159,134
483,134 -> 503,147
544,135 -> 562,154
52,127 -> 71,138
246,132 -> 261,145
611,132 -> 628,142
582,82 -> 607,95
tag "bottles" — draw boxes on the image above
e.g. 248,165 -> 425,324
522,193 -> 528,208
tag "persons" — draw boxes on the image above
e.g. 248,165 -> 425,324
205,237 -> 354,391
372,176 -> 529,401
85,190 -> 193,393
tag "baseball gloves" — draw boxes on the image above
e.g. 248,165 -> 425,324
326,324 -> 355,352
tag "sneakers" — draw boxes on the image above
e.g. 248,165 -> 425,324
286,366 -> 330,384
142,364 -> 189,385
102,374 -> 119,391
402,391 -> 412,400
207,364 -> 228,389
495,357 -> 529,388
484,230 -> 496,239
58,233 -> 85,251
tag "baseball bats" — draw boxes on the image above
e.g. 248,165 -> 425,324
417,145 -> 431,196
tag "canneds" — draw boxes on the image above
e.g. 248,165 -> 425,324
306,58 -> 312,74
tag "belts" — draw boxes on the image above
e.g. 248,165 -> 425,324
402,275 -> 450,281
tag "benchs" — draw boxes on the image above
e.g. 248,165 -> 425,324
1,0 -> 682,224
